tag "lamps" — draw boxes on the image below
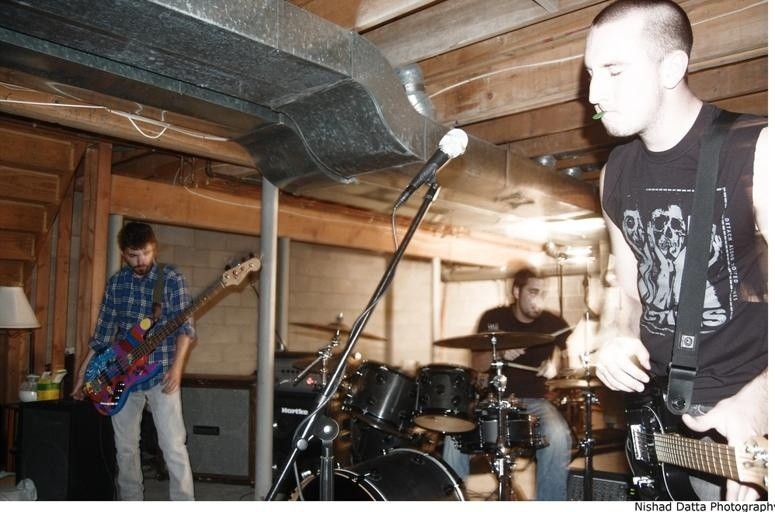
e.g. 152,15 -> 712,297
0,286 -> 42,374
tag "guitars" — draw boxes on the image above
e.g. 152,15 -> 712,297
623,404 -> 767,502
81,258 -> 262,417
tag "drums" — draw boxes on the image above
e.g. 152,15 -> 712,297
289,449 -> 467,502
418,363 -> 477,435
341,360 -> 416,439
460,411 -> 541,453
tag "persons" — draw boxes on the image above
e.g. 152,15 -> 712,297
70,221 -> 197,501
472,268 -> 572,501
582,1 -> 768,501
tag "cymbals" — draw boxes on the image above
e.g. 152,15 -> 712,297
433,331 -> 558,348
542,376 -> 601,390
293,355 -> 362,375
290,318 -> 385,342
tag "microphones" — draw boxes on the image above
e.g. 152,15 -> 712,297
393,129 -> 469,210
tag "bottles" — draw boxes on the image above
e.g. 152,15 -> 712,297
18,374 -> 39,401
